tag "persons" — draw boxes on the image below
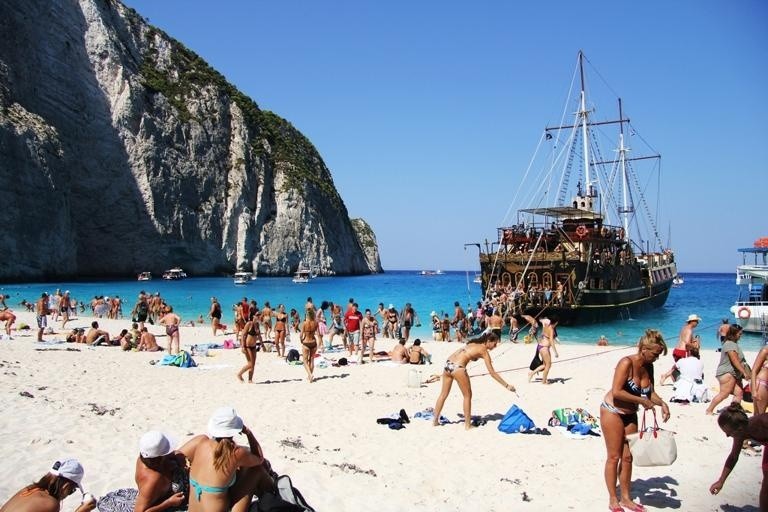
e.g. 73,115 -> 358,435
0,457 -> 97,512
188,295 -> 432,386
709,403 -> 768,512
659,312 -> 710,403
500,226 -> 673,267
133,430 -> 210,512
188,406 -> 276,512
0,288 -> 181,357
427,282 -> 568,431
704,317 -> 750,416
600,329 -> 670,512
742,347 -> 768,453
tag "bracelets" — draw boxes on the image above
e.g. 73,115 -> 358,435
247,431 -> 251,435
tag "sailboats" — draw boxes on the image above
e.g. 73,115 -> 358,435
468,50 -> 682,321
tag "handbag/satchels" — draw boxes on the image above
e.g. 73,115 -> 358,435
531,353 -> 541,372
626,429 -> 677,465
498,404 -> 536,435
249,475 -> 313,511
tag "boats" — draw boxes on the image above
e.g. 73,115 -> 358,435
162,267 -> 187,281
672,273 -> 685,286
137,270 -> 154,281
727,237 -> 767,335
251,275 -> 257,280
290,266 -> 319,284
418,267 -> 447,276
472,272 -> 482,285
233,267 -> 252,284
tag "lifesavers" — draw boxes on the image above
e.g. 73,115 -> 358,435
738,308 -> 750,319
601,227 -> 611,238
576,226 -> 586,236
619,228 -> 624,240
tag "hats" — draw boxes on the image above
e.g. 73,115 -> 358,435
50,458 -> 83,494
686,315 -> 701,322
140,433 -> 177,460
207,407 -> 243,439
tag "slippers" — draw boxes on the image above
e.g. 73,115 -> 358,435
624,503 -> 648,512
608,505 -> 624,511
